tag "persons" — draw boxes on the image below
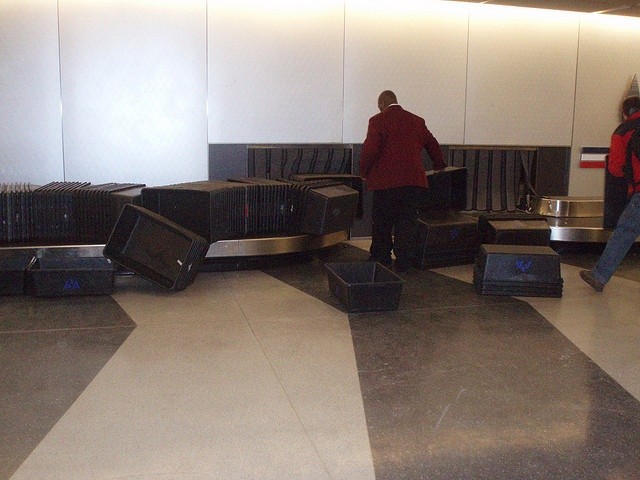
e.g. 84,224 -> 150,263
580,96 -> 640,292
359,90 -> 447,272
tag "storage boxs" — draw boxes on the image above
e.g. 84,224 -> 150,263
408,212 -> 564,298
102,203 -> 208,292
0,256 -> 36,296
0,181 -> 146,246
141,176 -> 359,245
323,261 -> 406,312
28,257 -> 115,296
425,166 -> 467,211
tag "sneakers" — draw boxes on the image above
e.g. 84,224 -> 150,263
580,270 -> 604,291
